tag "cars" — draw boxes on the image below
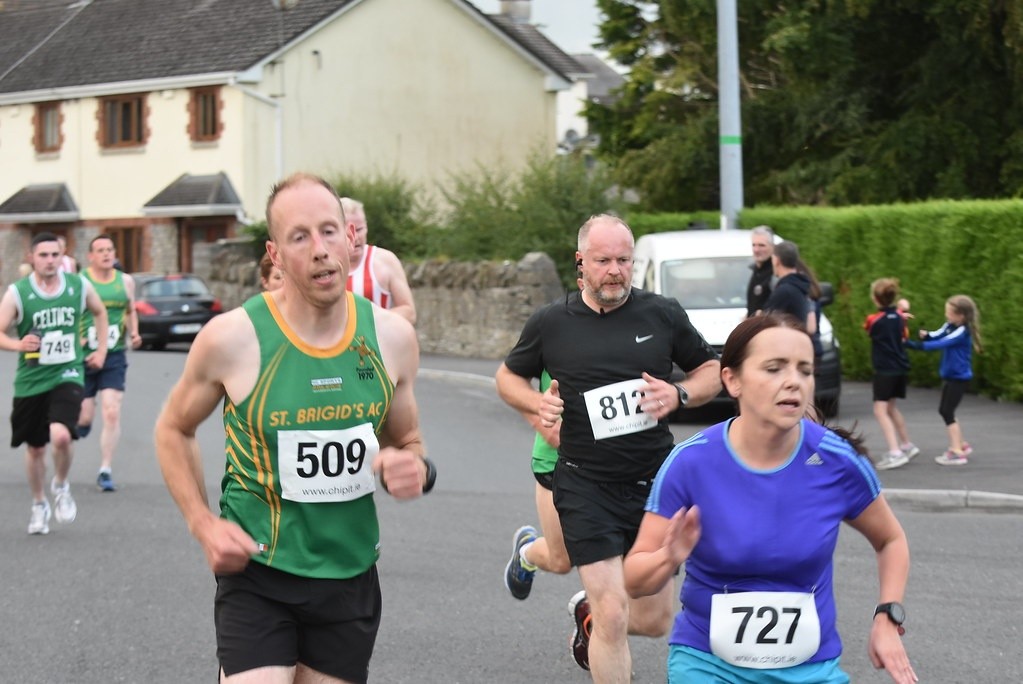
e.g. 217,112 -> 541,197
127,271 -> 225,350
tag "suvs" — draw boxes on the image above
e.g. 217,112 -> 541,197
637,229 -> 845,415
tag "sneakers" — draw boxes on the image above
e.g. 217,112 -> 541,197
962,442 -> 972,455
27,499 -> 51,533
568,589 -> 592,672
97,473 -> 115,491
50,476 -> 76,524
876,453 -> 909,469
902,443 -> 920,459
505,526 -> 538,600
77,425 -> 91,436
935,451 -> 968,464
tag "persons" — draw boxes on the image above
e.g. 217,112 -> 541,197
504,369 -> 572,601
153,172 -> 429,684
903,295 -> 982,468
621,310 -> 918,684
745,225 -> 824,427
258,253 -> 284,292
341,197 -> 417,328
493,213 -> 726,683
55,236 -> 78,276
0,232 -> 108,533
860,279 -> 921,471
78,233 -> 141,491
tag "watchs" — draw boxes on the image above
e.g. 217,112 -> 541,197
420,456 -> 437,494
673,382 -> 689,409
873,602 -> 906,625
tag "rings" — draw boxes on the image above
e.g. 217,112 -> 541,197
657,400 -> 665,407
